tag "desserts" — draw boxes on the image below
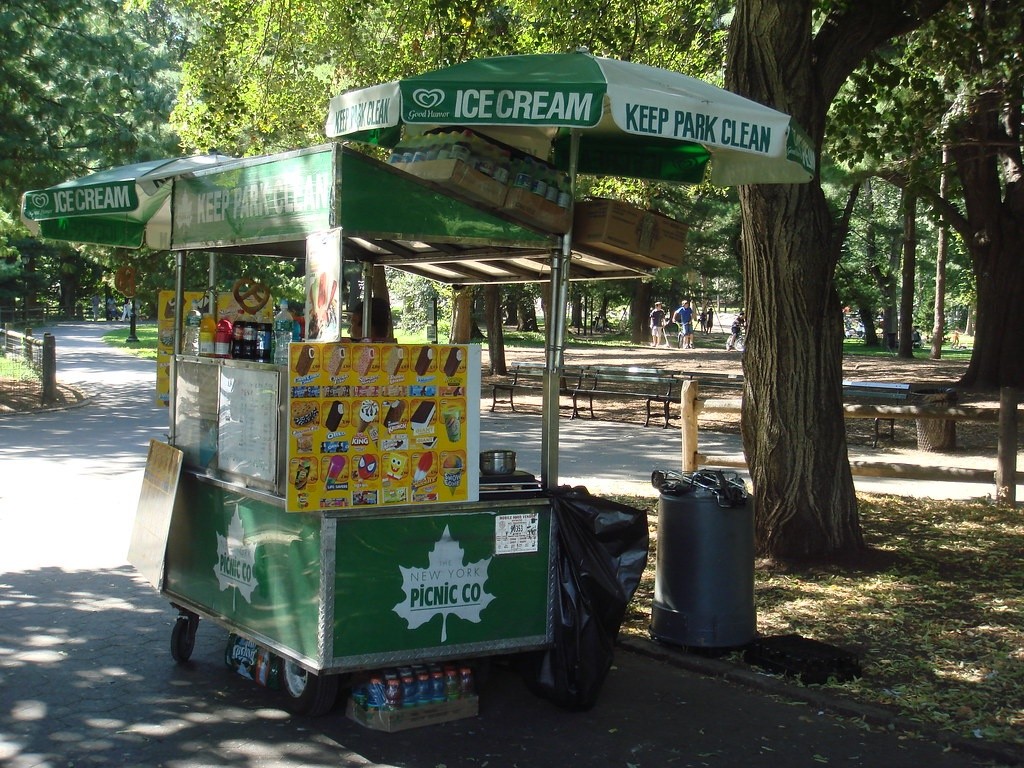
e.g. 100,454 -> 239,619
292,402 -> 319,427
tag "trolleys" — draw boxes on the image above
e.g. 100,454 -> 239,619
19,51 -> 816,714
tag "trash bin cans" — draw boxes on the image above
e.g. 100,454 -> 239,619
653,466 -> 759,655
907,387 -> 956,453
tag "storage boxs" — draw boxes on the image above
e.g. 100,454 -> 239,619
345,697 -> 479,733
499,188 -> 573,236
573,194 -> 689,269
391,159 -> 509,209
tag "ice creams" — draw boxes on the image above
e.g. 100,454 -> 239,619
358,399 -> 379,432
438,456 -> 464,495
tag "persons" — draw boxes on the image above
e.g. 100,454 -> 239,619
92,294 -> 102,322
107,296 -> 118,321
346,297 -> 390,338
700,308 -> 713,333
119,297 -> 131,322
728,311 -> 744,351
650,302 -> 665,348
622,308 -> 628,321
672,300 -> 695,348
951,327 -> 963,349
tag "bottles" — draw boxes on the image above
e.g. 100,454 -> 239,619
243,310 -> 257,361
254,309 -> 272,363
270,299 -> 305,365
214,314 -> 233,358
458,668 -> 475,699
255,646 -> 283,690
231,310 -> 247,360
199,313 -> 216,357
183,300 -> 201,357
347,661 -> 459,712
387,129 -> 572,212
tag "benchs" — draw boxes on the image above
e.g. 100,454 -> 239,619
485,362 -> 957,450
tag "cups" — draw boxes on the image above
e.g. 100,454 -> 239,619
444,407 -> 461,442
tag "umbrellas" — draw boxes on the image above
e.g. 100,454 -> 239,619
325,46 -> 817,490
19,154 -> 237,449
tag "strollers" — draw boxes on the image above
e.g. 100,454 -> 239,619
106,304 -> 120,321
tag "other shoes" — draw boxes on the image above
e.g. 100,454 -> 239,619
119,319 -> 124,323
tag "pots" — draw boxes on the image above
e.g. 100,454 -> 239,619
479,450 -> 515,475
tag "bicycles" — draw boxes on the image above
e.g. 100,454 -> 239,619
673,320 -> 690,348
725,324 -> 746,352
